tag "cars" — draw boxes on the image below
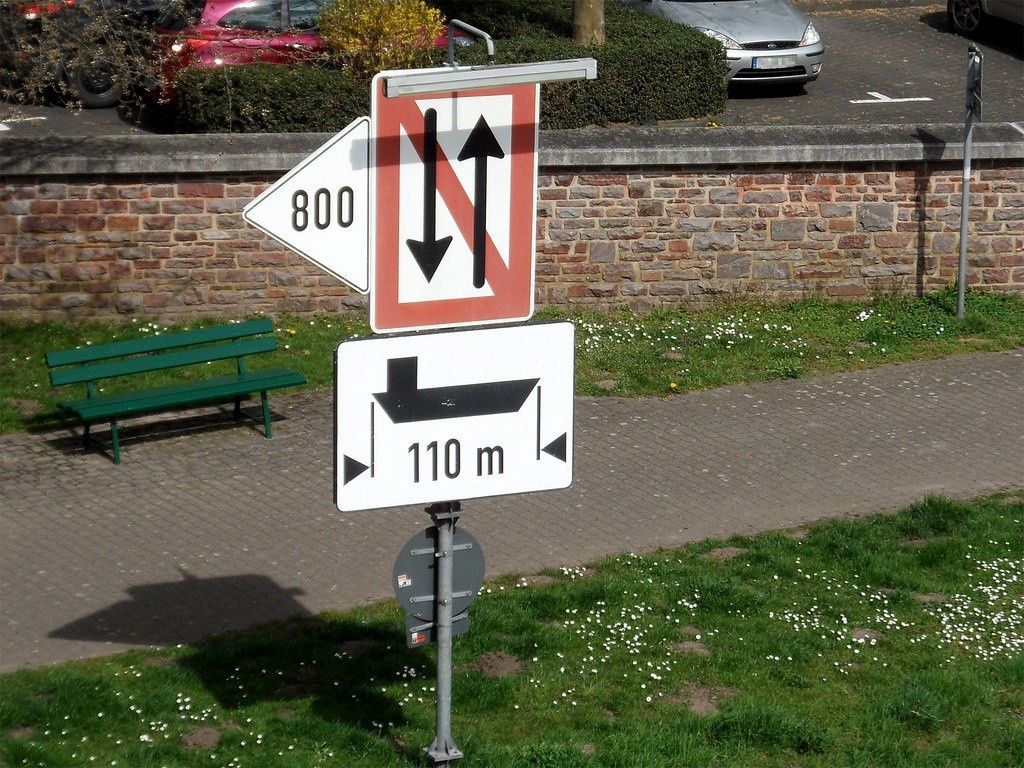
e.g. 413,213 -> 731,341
16,0 -> 478,131
621,0 -> 823,89
948,0 -> 1024,38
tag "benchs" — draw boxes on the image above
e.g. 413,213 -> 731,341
45,317 -> 308,464
